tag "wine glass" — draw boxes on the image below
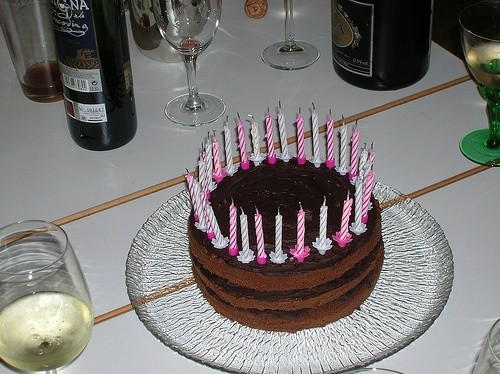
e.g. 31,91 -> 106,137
259,0 -> 321,69
455,9 -> 500,171
0,219 -> 93,374
152,0 -> 227,127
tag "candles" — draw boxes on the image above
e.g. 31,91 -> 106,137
185,101 -> 375,264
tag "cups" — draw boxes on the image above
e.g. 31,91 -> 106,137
1,0 -> 66,103
471,316 -> 500,374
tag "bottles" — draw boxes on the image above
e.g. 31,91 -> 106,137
329,0 -> 434,91
129,0 -> 202,63
52,0 -> 137,152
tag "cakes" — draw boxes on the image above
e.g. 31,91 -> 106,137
186,157 -> 385,333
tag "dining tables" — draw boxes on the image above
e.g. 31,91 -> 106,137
0,0 -> 500,374
